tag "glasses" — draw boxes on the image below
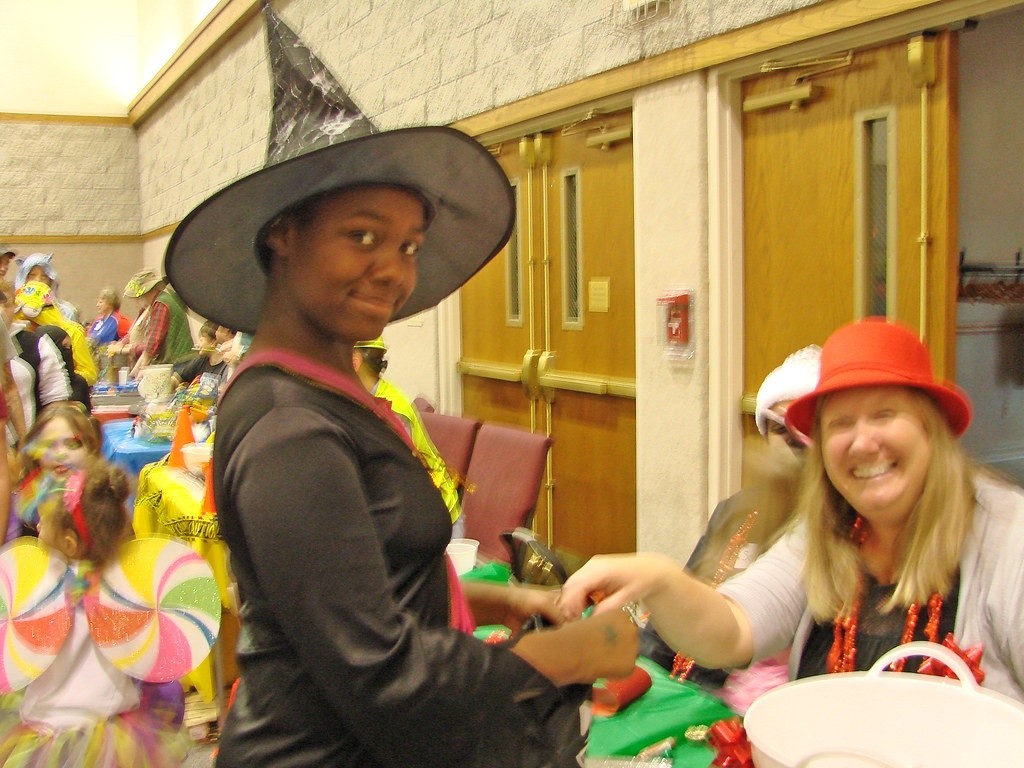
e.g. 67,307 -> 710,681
767,422 -> 786,434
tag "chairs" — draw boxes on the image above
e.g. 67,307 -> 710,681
410,397 -> 434,420
419,412 -> 484,492
452,423 -> 554,567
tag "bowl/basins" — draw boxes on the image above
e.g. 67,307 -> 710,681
181,446 -> 211,477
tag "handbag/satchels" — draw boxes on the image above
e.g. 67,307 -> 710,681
506,542 -> 588,586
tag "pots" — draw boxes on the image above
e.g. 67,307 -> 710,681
743,641 -> 1024,768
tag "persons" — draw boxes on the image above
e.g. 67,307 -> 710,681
641,343 -> 822,691
562,320 -> 1024,705
215,151 -> 641,768
0,245 -> 247,768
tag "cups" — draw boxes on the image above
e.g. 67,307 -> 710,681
121,367 -> 130,374
446,544 -> 476,576
451,539 -> 479,566
119,370 -> 127,385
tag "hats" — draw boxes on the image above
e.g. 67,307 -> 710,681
123,271 -> 164,299
756,344 -> 819,434
0,247 -> 17,260
785,320 -> 971,440
164,6 -> 516,333
354,333 -> 388,351
97,289 -> 119,306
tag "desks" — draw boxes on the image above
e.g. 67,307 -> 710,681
90,405 -> 131,432
93,380 -> 140,413
102,421 -> 169,520
